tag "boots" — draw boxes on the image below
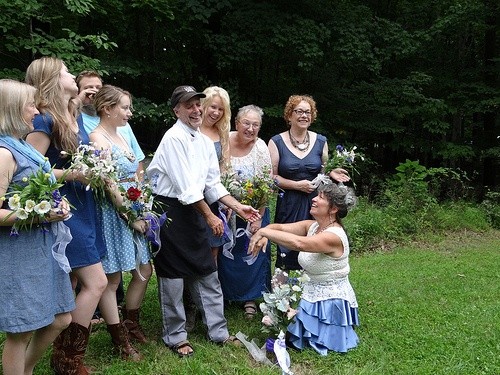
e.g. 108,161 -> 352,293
107,319 -> 144,362
50,328 -> 66,365
122,305 -> 150,344
56,321 -> 91,375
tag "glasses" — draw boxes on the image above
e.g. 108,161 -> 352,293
293,109 -> 313,116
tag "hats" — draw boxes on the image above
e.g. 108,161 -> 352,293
170,86 -> 207,109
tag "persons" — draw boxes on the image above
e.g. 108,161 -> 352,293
269,96 -> 351,284
183,86 -> 231,333
0,79 -> 76,375
25,56 -> 165,375
247,184 -> 360,357
217,105 -> 274,320
145,86 -> 263,356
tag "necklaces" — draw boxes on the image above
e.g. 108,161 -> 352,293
288,128 -> 310,152
313,220 -> 336,236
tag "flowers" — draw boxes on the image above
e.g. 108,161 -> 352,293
220,165 -> 284,213
262,265 -> 314,338
312,145 -> 365,191
0,140 -> 168,235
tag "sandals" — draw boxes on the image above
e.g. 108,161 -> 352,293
244,301 -> 257,318
165,340 -> 194,356
211,336 -> 244,347
